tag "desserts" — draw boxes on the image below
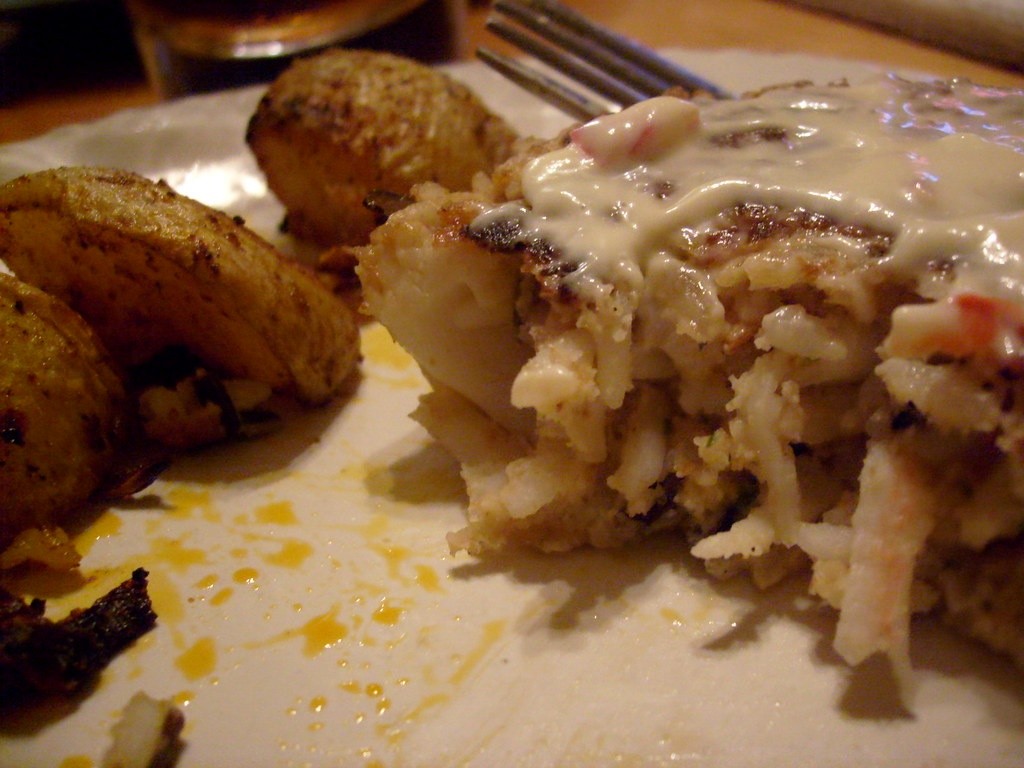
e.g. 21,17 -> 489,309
352,79 -> 1024,707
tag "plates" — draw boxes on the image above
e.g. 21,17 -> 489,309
0,48 -> 1024,768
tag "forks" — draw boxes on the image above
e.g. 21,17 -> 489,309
473,0 -> 741,124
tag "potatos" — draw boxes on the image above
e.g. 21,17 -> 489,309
0,46 -> 503,555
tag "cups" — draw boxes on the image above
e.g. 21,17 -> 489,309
129,0 -> 460,106
